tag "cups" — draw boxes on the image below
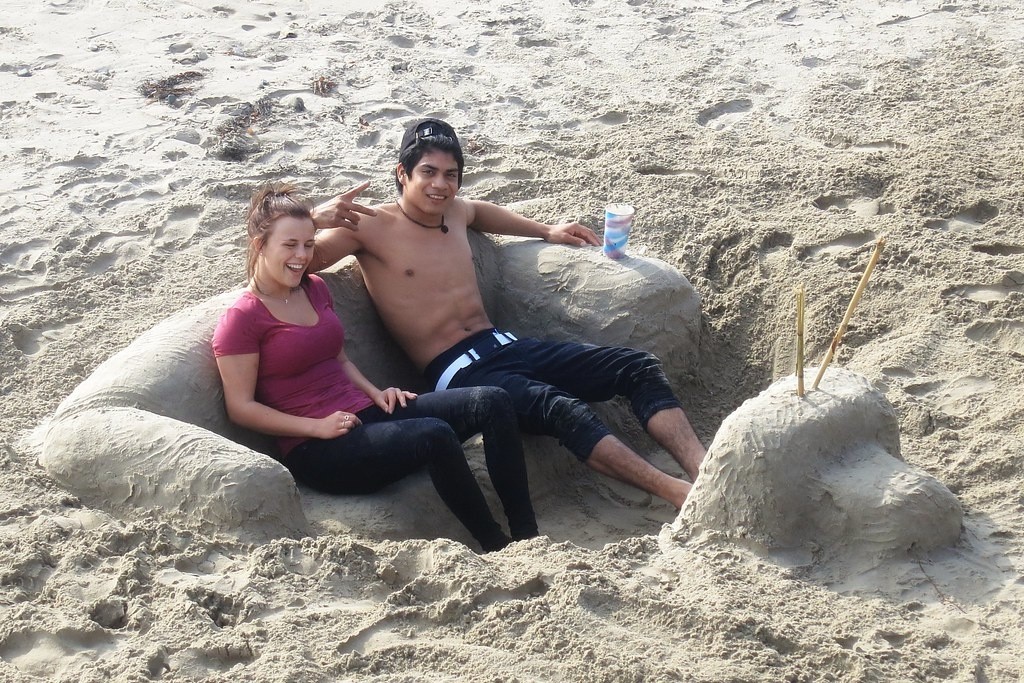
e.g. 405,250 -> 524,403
603,203 -> 635,258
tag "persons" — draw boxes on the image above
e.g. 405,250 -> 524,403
211,178 -> 541,552
304,117 -> 708,507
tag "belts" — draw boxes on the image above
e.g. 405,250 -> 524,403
434,331 -> 521,392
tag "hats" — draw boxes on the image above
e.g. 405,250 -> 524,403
400,117 -> 461,154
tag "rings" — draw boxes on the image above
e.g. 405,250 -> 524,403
341,421 -> 345,428
344,414 -> 350,421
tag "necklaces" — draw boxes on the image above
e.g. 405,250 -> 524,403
250,284 -> 291,304
395,199 -> 449,234
